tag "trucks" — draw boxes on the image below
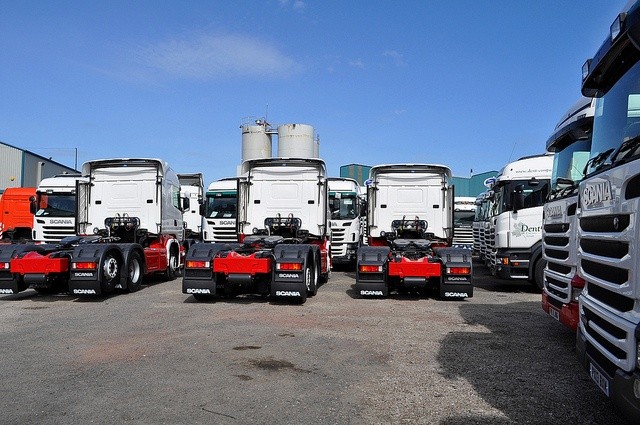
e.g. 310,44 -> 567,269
181,157 -> 332,303
326,177 -> 367,270
0,158 -> 189,297
452,197 -> 475,249
356,164 -> 472,299
472,151 -> 587,293
29,172 -> 88,244
574,0 -> 640,425
0,187 -> 36,242
178,173 -> 204,236
540,94 -> 640,330
202,177 -> 238,243
